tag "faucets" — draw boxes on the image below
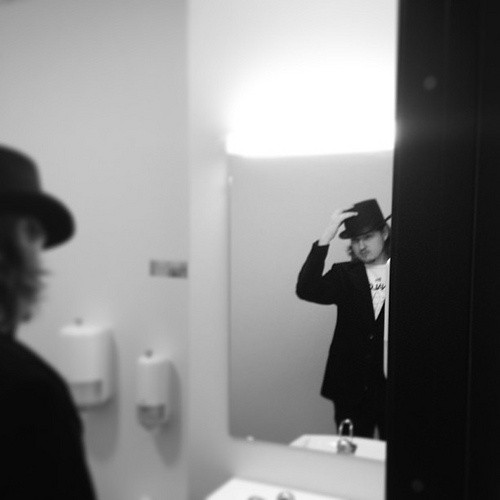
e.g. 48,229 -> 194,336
335,419 -> 358,455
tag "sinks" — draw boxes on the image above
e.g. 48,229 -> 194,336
205,476 -> 347,500
288,432 -> 386,460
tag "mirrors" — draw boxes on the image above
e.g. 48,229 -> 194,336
228,3 -> 400,460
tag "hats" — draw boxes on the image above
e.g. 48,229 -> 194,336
1,145 -> 74,251
337,199 -> 390,238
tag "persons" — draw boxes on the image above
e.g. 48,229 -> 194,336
296,199 -> 392,442
0,145 -> 102,500
